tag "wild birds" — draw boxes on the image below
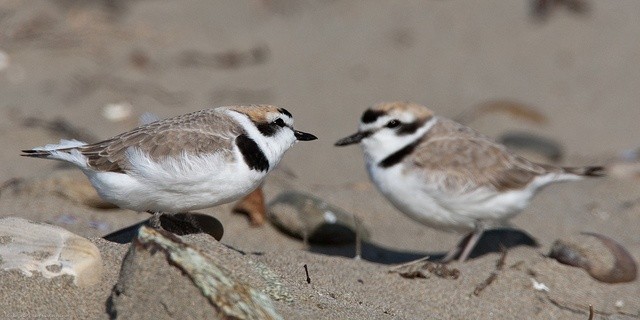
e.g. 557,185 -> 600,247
20,103 -> 318,244
334,101 -> 606,279
